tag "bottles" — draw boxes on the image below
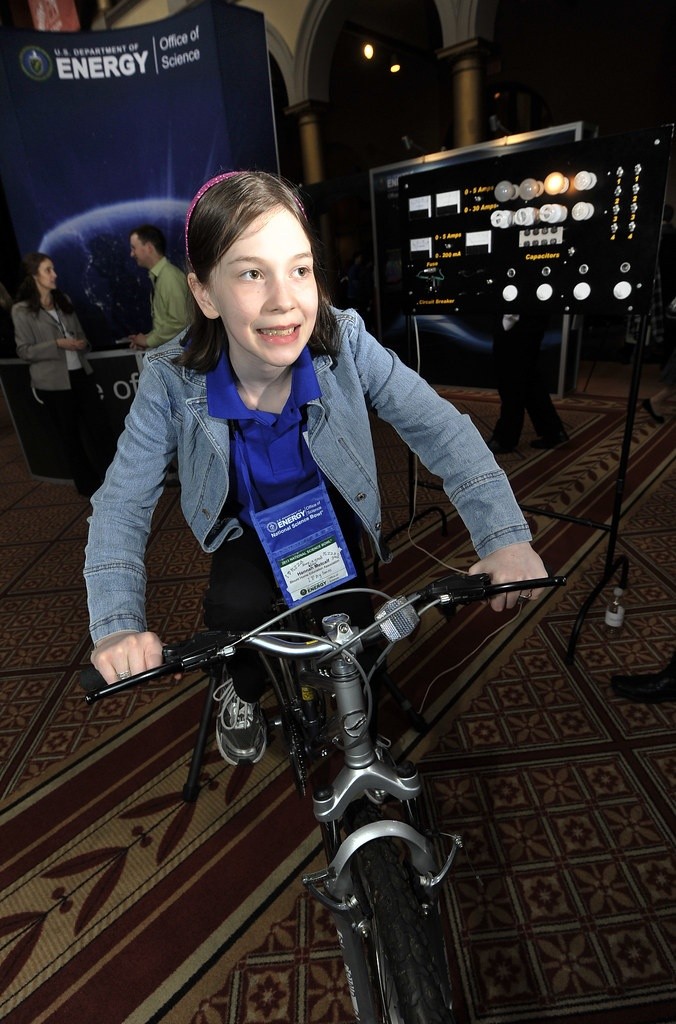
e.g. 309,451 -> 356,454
605,588 -> 624,640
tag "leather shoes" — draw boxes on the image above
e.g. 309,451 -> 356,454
611,654 -> 676,703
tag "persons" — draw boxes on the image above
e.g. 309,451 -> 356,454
613,651 -> 676,705
82,172 -> 574,768
123,225 -> 195,494
11,252 -> 110,498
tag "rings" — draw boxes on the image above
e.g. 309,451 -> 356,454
117,672 -> 132,681
518,591 -> 531,604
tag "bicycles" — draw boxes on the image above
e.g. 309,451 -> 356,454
77,564 -> 572,1024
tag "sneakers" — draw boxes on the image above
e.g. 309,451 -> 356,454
364,734 -> 395,804
213,664 -> 267,765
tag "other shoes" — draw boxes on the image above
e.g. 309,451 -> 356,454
489,432 -> 519,452
530,430 -> 570,450
79,485 -> 99,497
